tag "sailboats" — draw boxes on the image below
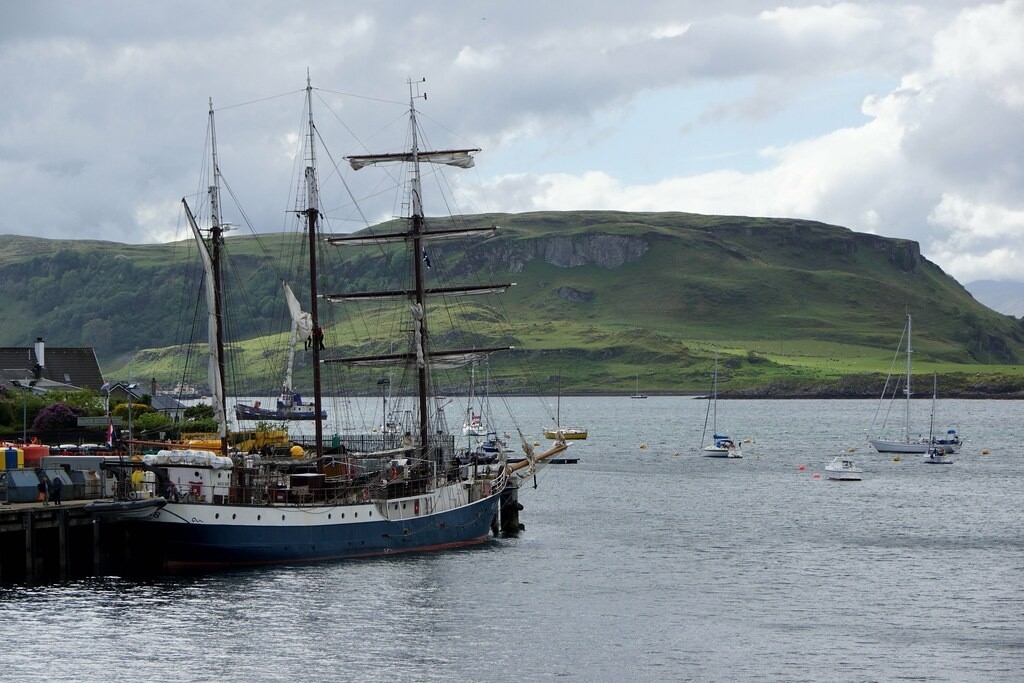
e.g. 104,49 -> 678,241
698,343 -> 744,458
864,301 -> 963,454
630,372 -> 649,399
96,65 -> 574,568
922,370 -> 955,464
541,365 -> 589,440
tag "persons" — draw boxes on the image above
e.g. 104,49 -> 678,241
41,477 -> 51,505
303,323 -> 326,350
166,481 -> 179,502
53,475 -> 64,505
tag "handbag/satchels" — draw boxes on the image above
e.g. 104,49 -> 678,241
39,492 -> 45,500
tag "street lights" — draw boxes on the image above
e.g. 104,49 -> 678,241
11,380 -> 38,444
376,376 -> 390,448
126,382 -> 140,480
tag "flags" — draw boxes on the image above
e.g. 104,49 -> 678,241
107,418 -> 116,450
422,246 -> 433,270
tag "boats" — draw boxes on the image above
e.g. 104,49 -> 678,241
823,449 -> 864,481
82,492 -> 168,521
